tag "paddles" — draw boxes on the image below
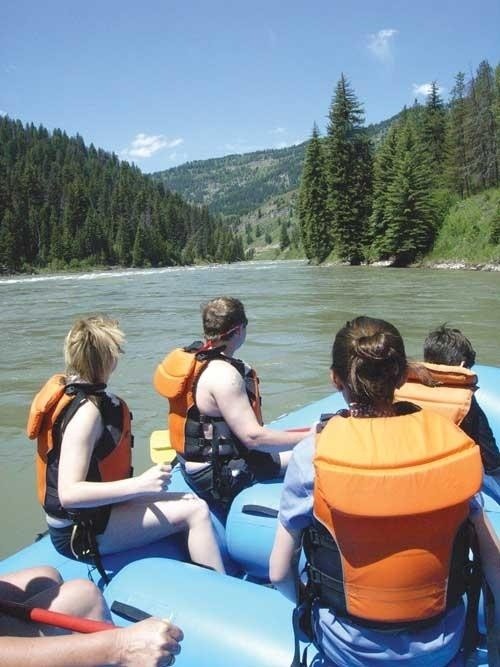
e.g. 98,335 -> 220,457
149,428 -> 312,465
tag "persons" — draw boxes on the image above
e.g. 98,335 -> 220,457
154,296 -> 321,512
26,313 -> 227,576
0,566 -> 184,667
269,315 -> 500,667
391,321 -> 499,476
